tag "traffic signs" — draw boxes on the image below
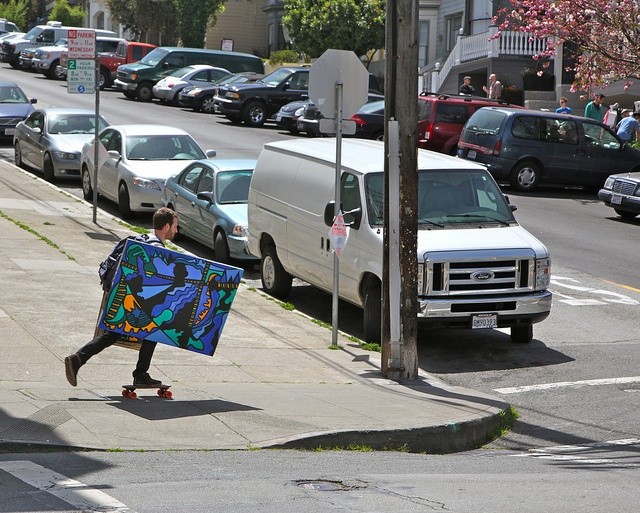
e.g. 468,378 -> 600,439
67,58 -> 96,92
327,210 -> 347,258
68,29 -> 96,58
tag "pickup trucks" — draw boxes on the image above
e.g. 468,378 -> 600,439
57,41 -> 158,88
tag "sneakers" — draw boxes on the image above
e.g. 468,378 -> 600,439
132,371 -> 163,386
64,353 -> 80,386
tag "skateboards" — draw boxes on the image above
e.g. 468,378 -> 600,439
122,384 -> 172,399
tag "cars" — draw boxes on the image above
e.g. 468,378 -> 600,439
0,81 -> 37,138
352,100 -> 385,139
276,93 -> 311,135
161,159 -> 261,261
19,39 -> 66,79
152,64 -> 233,105
598,171 -> 640,216
13,108 -> 110,180
81,124 -> 216,217
296,104 -> 322,135
179,71 -> 266,112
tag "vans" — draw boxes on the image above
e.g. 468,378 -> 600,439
114,46 -> 264,100
456,106 -> 639,191
0,20 -> 117,69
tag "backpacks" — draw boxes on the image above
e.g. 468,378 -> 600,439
98,233 -> 164,290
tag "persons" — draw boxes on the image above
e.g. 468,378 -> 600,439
616,110 -> 640,144
65,208 -> 179,388
555,96 -> 573,115
459,76 -> 475,95
482,74 -> 502,100
584,92 -> 608,124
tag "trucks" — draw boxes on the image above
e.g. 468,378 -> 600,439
248,137 -> 551,340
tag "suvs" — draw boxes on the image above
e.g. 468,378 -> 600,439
417,93 -> 527,155
213,66 -> 382,125
32,36 -> 123,78
